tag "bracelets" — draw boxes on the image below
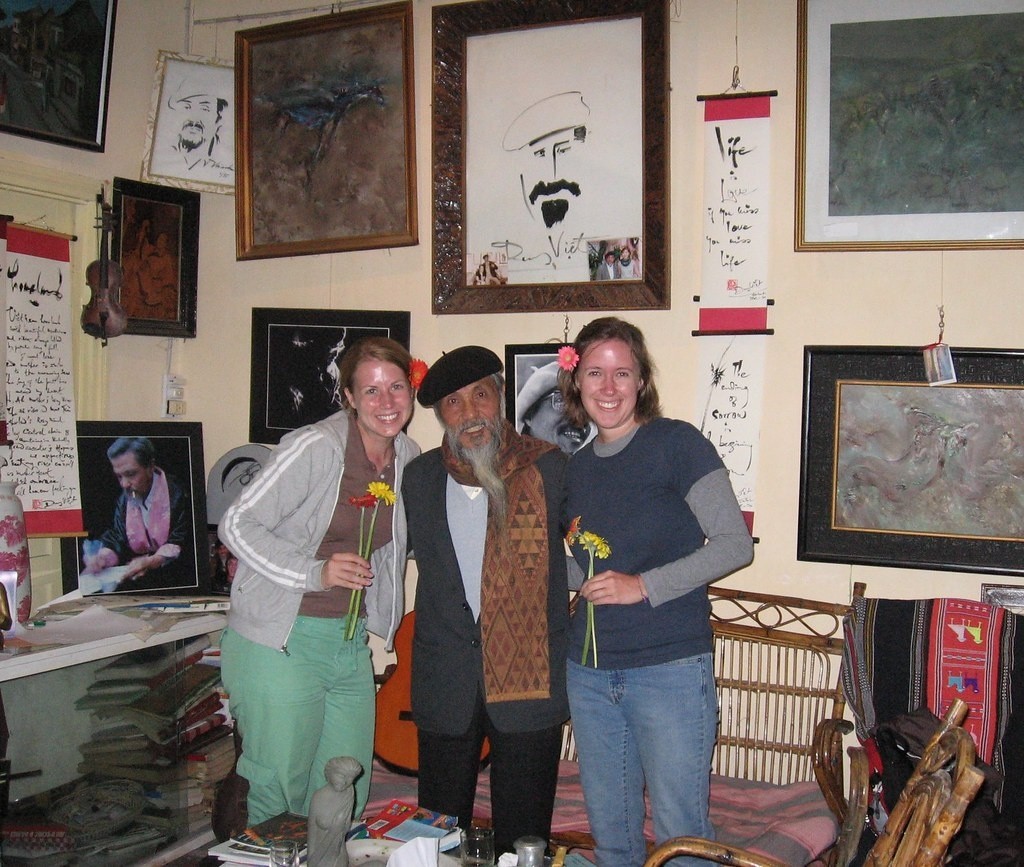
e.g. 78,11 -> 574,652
634,574 -> 648,603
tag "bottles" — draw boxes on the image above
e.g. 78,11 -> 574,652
513,835 -> 547,867
0,482 -> 32,625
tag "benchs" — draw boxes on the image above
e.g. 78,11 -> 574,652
362,581 -> 869,867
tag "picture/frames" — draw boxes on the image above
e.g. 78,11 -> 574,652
146,50 -> 237,197
249,305 -> 411,446
108,177 -> 201,339
0,0 -> 119,153
61,419 -> 211,603
502,343 -> 587,455
430,0 -> 670,313
795,342 -> 1024,578
793,0 -> 1024,253
233,0 -> 417,262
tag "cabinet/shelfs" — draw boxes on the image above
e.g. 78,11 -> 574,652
0,613 -> 231,867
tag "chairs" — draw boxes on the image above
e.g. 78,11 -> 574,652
645,695 -> 987,867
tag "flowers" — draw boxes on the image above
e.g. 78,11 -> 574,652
565,514 -> 612,671
343,480 -> 398,642
555,346 -> 580,373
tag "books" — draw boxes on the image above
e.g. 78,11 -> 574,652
0,820 -> 76,867
366,800 -> 462,852
135,600 -> 230,612
208,811 -> 308,867
74,635 -> 237,853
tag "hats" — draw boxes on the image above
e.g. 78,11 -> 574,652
417,345 -> 504,408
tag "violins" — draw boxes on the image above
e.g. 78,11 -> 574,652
79,202 -> 128,340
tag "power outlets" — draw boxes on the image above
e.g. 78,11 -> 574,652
160,373 -> 187,419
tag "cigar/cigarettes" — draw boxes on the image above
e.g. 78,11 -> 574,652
132,490 -> 136,498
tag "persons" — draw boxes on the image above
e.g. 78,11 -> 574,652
518,360 -> 593,455
83,437 -> 196,591
306,757 -> 361,867
272,327 -> 347,428
559,316 -> 755,867
597,247 -> 641,281
217,338 -> 421,830
209,538 -> 239,591
475,255 -> 501,284
400,345 -> 571,867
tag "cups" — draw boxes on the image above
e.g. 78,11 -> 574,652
460,827 -> 495,867
269,840 -> 299,867
